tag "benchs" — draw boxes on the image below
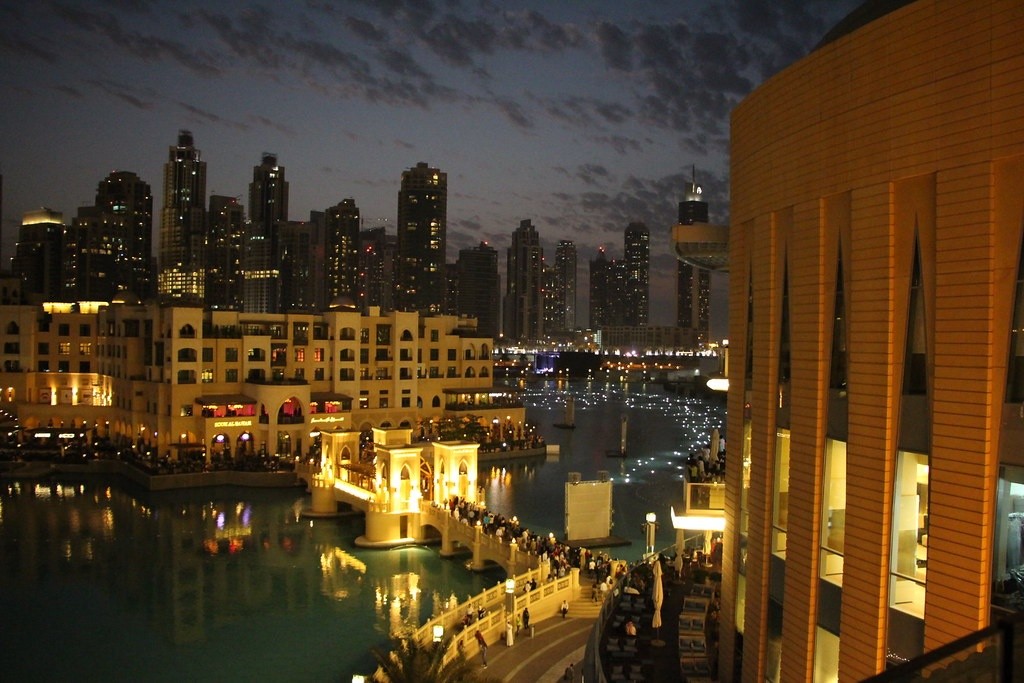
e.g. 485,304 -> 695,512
679,657 -> 712,676
682,596 -> 709,614
678,636 -> 707,651
679,614 -> 706,635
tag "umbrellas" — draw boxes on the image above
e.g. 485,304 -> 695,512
652,560 -> 663,641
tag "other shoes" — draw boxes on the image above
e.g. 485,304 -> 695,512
481,664 -> 488,669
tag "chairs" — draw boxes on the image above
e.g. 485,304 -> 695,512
606,662 -> 629,680
624,636 -> 642,655
634,597 -> 646,615
604,635 -> 621,653
629,663 -> 646,681
610,614 -> 625,631
629,614 -> 640,633
619,593 -> 632,613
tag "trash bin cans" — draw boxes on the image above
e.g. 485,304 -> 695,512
529,624 -> 535,638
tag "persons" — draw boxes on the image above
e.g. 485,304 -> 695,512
475,631 -> 488,669
506,622 -> 514,646
524,434 -> 545,449
457,641 -> 466,665
463,603 -> 486,626
688,434 -> 727,505
564,664 -> 574,683
560,601 -> 569,618
523,608 -> 529,629
431,497 -> 627,605
517,614 -> 521,636
618,532 -> 722,651
